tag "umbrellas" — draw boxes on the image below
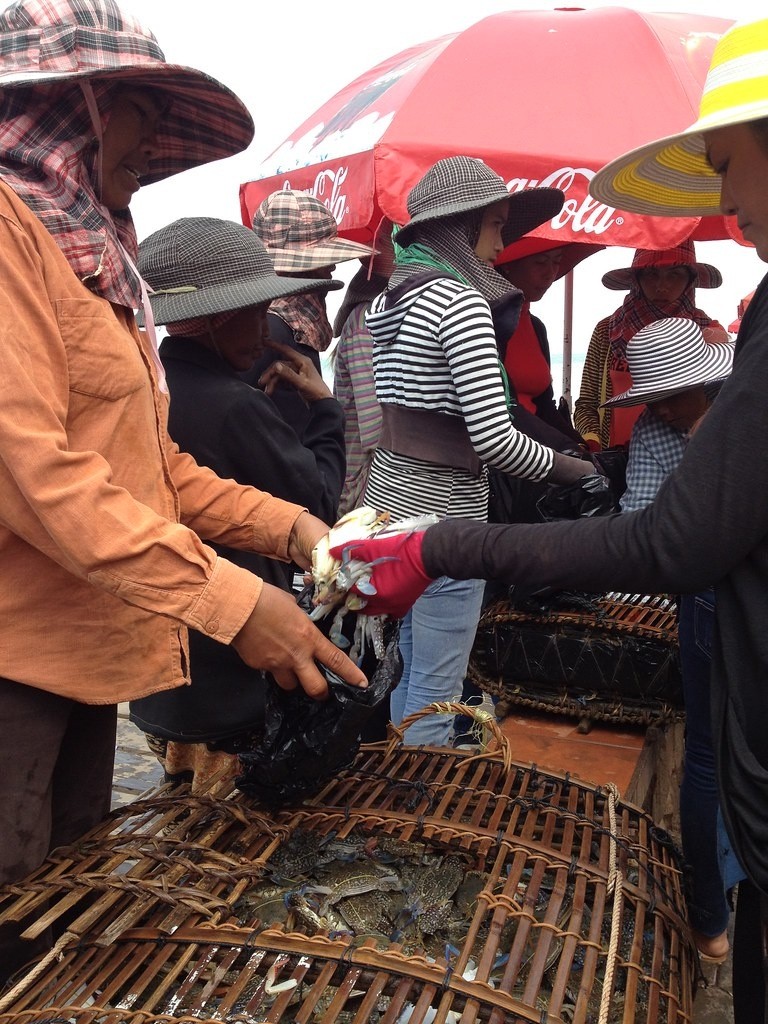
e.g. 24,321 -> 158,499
239,7 -> 756,420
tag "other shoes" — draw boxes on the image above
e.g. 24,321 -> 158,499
696,946 -> 730,963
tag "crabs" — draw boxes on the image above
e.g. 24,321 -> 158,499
86,811 -> 683,1024
304,505 -> 439,667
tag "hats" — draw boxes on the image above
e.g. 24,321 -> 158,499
590,16 -> 768,217
359,214 -> 396,279
252,189 -> 380,272
393,156 -> 565,247
601,238 -> 722,290
494,236 -> 573,265
0,0 -> 255,185
599,319 -> 738,409
133,218 -> 345,327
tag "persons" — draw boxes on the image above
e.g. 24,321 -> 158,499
571,237 -> 722,453
1,2 -> 370,991
327,223 -> 589,742
363,154 -> 563,747
136,215 -> 344,787
598,318 -> 745,964
329,16 -> 768,1024
251,187 -> 383,352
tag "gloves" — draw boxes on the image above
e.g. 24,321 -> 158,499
548,449 -> 597,485
329,530 -> 435,621
